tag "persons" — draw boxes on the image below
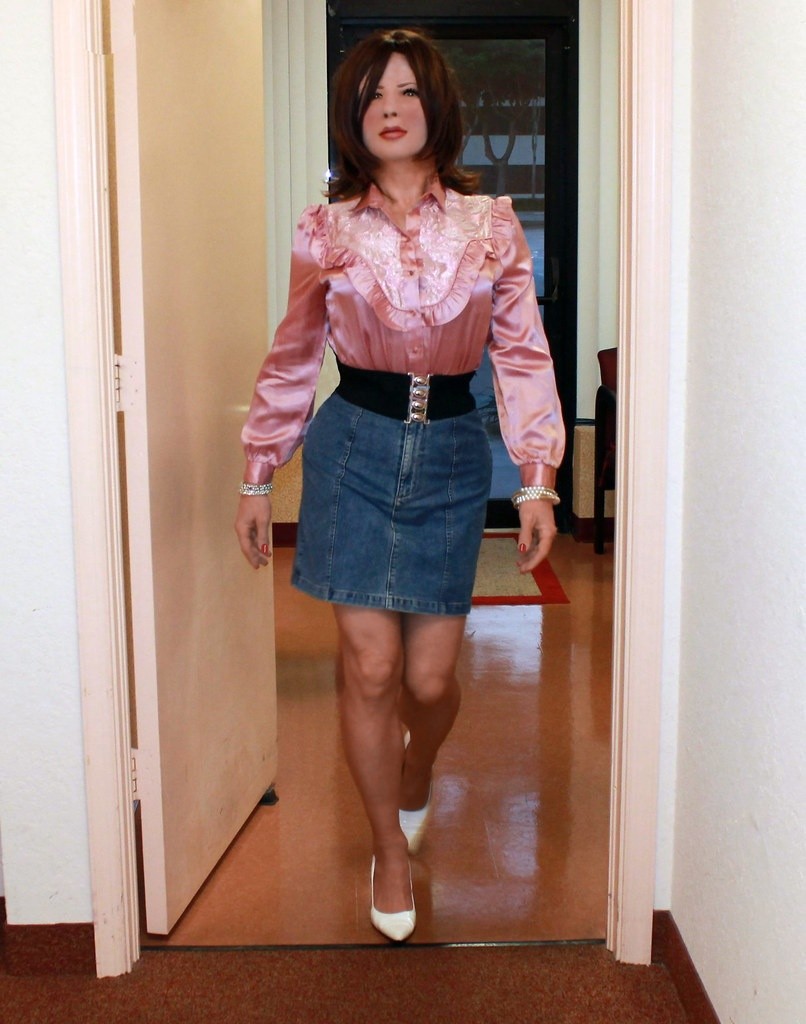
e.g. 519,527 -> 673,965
236,28 -> 569,942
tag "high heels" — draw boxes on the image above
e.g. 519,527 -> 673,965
399,730 -> 435,846
371,854 -> 416,941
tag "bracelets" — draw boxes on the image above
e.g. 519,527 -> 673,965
237,481 -> 274,495
511,486 -> 561,506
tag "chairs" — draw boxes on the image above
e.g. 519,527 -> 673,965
593,349 -> 617,557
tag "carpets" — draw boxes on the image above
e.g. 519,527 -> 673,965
461,531 -> 568,608
